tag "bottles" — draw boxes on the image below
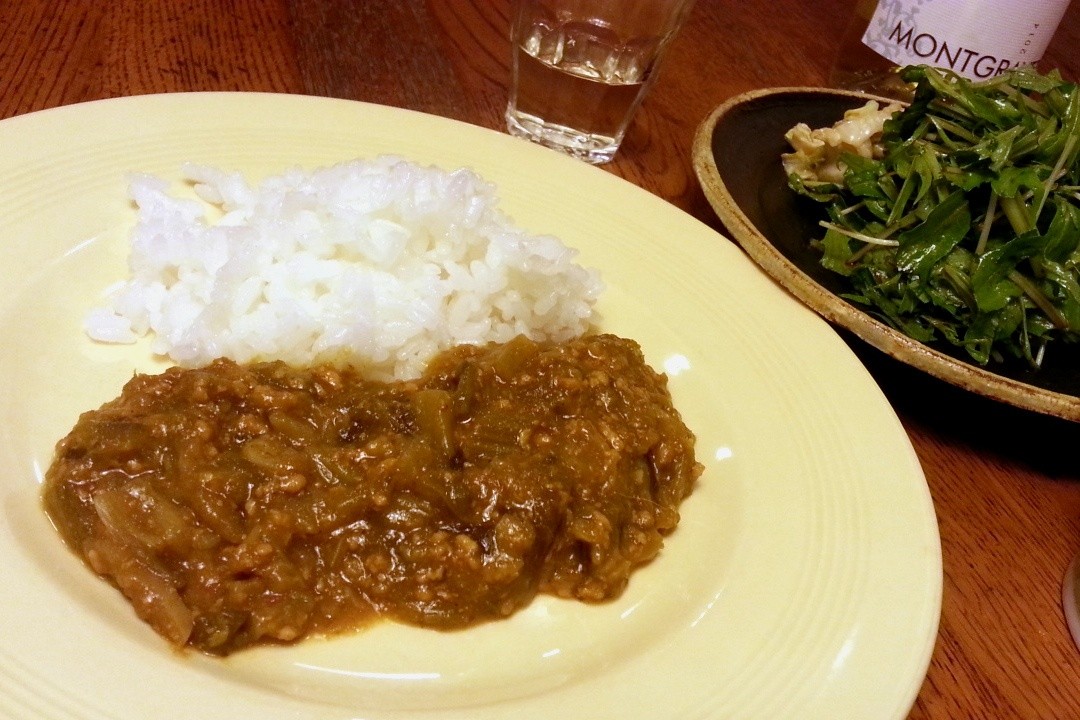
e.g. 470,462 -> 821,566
829,0 -> 1070,104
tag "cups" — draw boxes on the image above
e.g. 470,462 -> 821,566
505,0 -> 694,165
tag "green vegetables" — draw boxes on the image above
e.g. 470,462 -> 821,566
789,65 -> 1080,369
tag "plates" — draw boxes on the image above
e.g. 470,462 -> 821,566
0,92 -> 941,720
692,87 -> 1080,423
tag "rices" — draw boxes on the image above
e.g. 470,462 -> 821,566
81,161 -> 604,379
